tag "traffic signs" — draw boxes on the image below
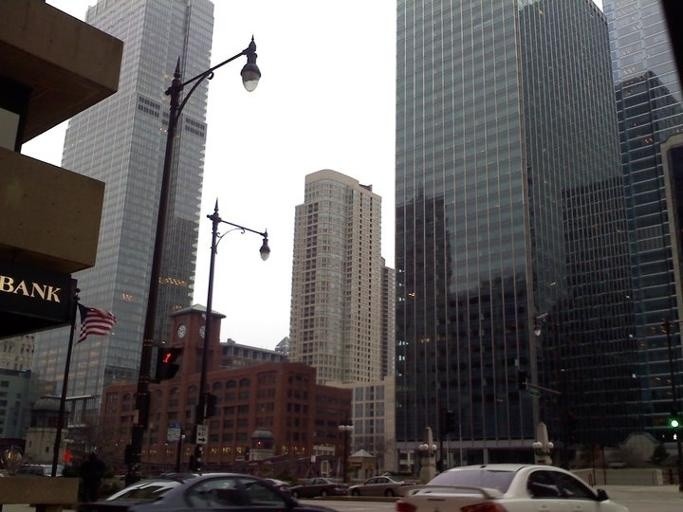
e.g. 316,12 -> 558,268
195,424 -> 208,444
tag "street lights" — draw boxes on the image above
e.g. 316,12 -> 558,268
338,418 -> 353,483
122,31 -> 262,483
187,196 -> 270,467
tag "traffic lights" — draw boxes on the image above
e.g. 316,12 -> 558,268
204,393 -> 217,417
669,417 -> 680,441
155,345 -> 183,381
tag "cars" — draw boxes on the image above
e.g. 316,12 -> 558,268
348,475 -> 417,496
20,463 -> 64,478
398,461 -> 629,512
100,471 -> 339,511
288,478 -> 350,495
265,478 -> 291,492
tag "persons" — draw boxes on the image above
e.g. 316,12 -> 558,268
76,474 -> 103,505
125,464 -> 141,488
587,471 -> 592,485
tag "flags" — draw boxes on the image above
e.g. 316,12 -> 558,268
78,302 -> 116,346
